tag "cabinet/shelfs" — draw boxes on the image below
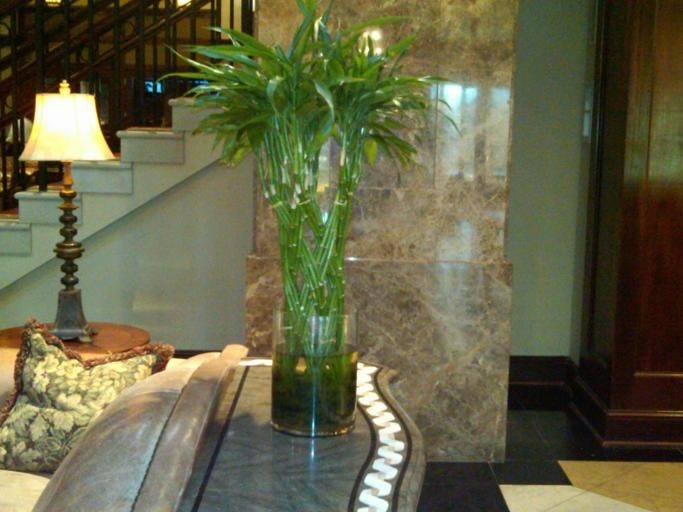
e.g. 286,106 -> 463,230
568,1 -> 683,452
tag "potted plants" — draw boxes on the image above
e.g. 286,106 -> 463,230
159,12 -> 463,439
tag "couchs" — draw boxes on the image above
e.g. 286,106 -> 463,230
1,340 -> 249,510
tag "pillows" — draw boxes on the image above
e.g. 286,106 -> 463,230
1,312 -> 175,474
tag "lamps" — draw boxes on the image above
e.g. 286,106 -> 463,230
18,81 -> 118,345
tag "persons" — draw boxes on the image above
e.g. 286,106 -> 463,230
4,117 -> 32,170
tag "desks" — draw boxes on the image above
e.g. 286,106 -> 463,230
1,323 -> 152,365
